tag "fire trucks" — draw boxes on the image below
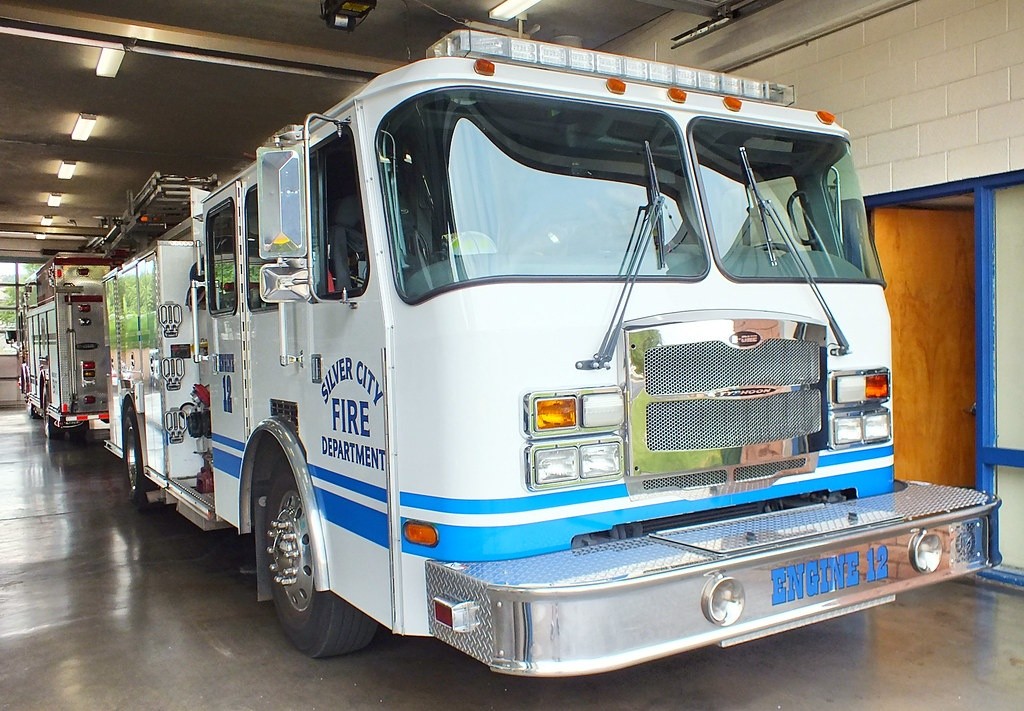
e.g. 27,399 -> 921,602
109,28 -> 1004,680
6,251 -> 104,444
